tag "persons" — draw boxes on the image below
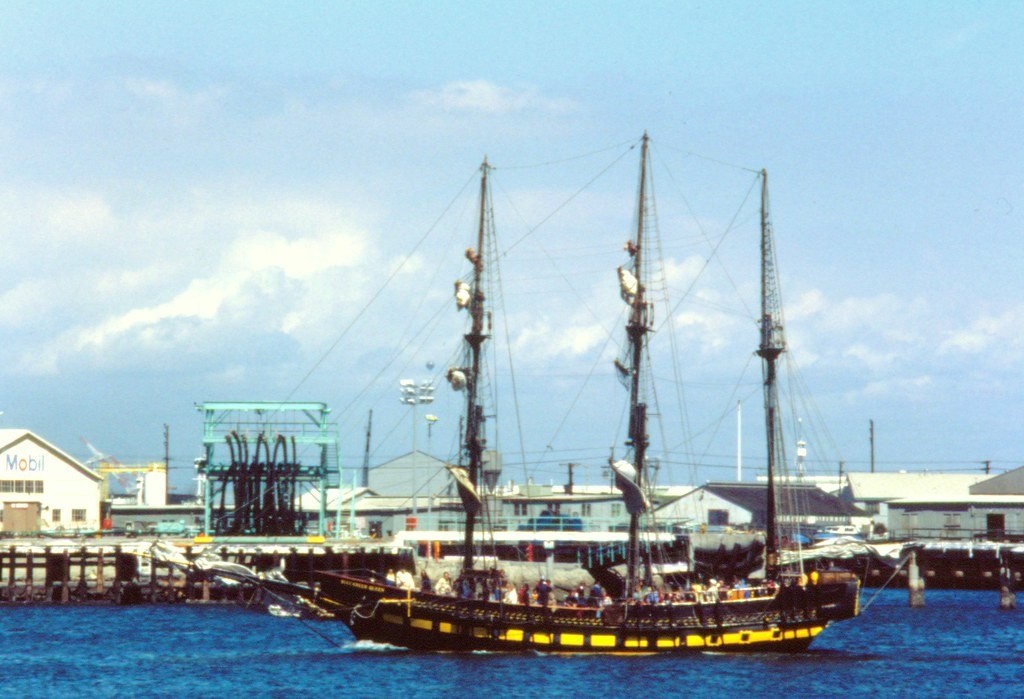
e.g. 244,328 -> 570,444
633,581 -> 663,603
386,568 -> 431,593
669,575 -> 750,602
562,580 -> 612,618
435,568 -> 502,602
503,576 -> 552,604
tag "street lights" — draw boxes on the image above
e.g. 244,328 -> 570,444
397,378 -> 440,531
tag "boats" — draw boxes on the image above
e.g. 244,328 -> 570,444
791,533 -> 811,546
813,525 -> 868,545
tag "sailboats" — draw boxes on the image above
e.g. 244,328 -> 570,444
121,129 -> 924,657
